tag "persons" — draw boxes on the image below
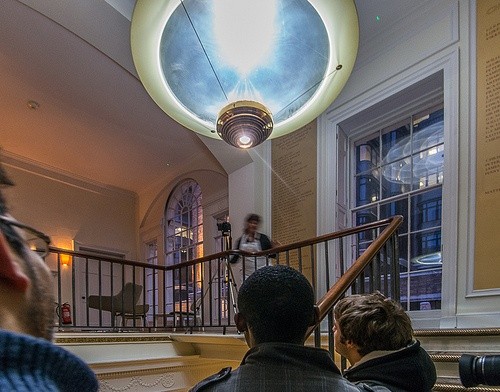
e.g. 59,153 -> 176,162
333,291 -> 436,392
0,194 -> 99,392
228,212 -> 281,289
188,265 -> 391,392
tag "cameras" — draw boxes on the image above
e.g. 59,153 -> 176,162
217,221 -> 231,232
458,354 -> 500,388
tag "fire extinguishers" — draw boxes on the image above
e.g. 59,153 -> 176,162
62,301 -> 72,324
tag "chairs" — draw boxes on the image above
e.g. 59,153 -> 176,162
89,282 -> 197,332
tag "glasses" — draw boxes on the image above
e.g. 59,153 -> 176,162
0,215 -> 51,259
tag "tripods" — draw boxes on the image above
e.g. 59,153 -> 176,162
185,231 -> 240,335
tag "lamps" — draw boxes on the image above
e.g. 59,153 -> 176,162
61,254 -> 69,265
130,0 -> 360,149
